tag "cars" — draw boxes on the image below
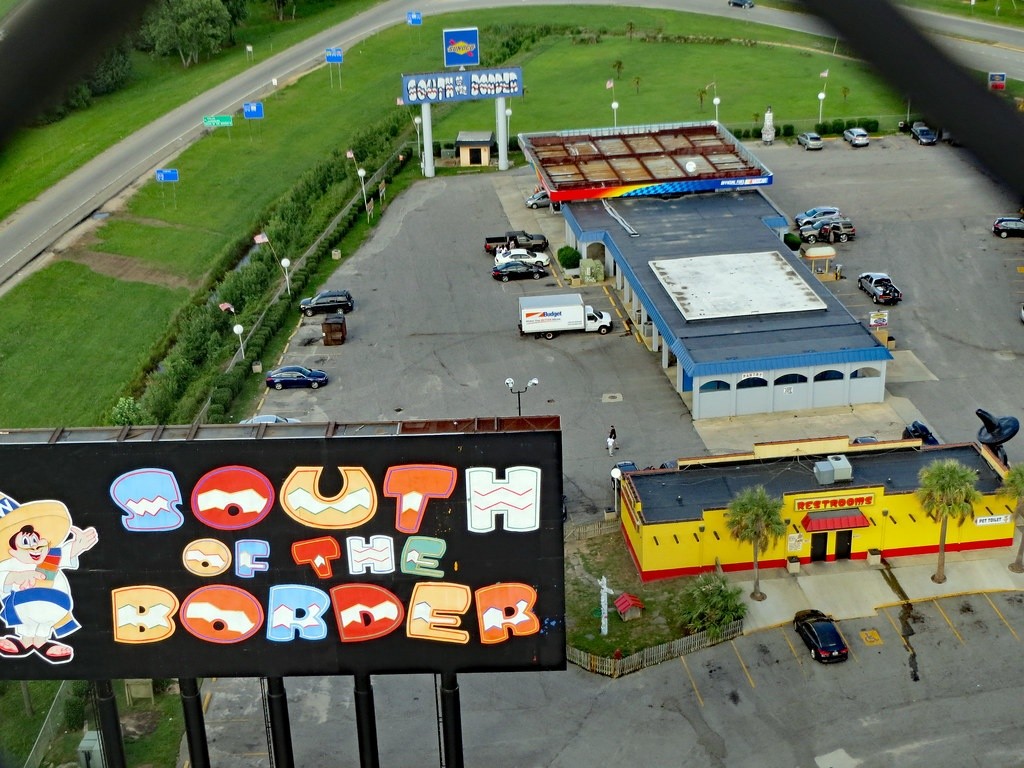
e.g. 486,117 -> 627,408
491,248 -> 551,282
910,126 -> 936,145
238,414 -> 301,425
265,364 -> 328,389
728,0 -> 754,9
525,189 -> 551,210
992,216 -> 1024,239
853,436 -> 878,444
792,608 -> 849,665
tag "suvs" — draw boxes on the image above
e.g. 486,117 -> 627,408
902,421 -> 939,446
799,217 -> 857,245
299,289 -> 355,318
797,131 -> 824,151
843,127 -> 869,148
795,206 -> 843,227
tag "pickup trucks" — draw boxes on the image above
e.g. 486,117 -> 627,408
484,230 -> 548,258
857,272 -> 902,306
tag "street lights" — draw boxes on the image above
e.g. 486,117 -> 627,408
611,102 -> 618,128
817,92 -> 825,124
357,168 -> 367,206
712,97 -> 720,121
414,116 -> 422,157
610,468 -> 622,512
505,108 -> 512,152
233,324 -> 246,360
505,377 -> 539,416
281,257 -> 291,296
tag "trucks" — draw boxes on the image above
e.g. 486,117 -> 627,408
517,292 -> 613,340
